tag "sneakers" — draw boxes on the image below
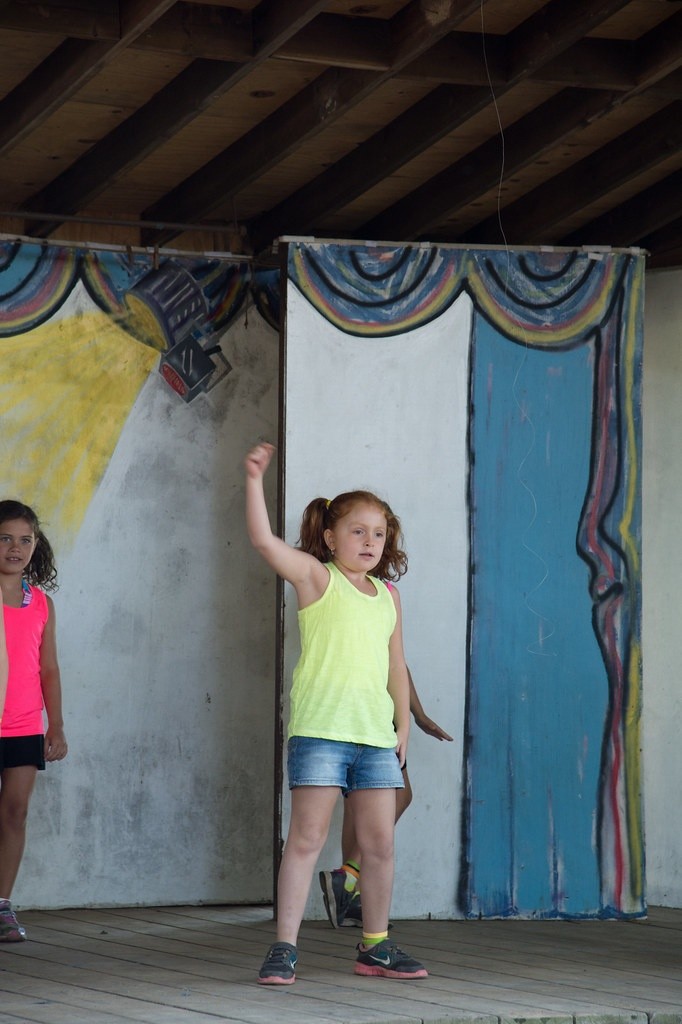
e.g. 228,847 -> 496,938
257,941 -> 299,985
0,899 -> 28,942
319,868 -> 394,930
354,937 -> 429,978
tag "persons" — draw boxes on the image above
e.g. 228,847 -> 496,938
319,659 -> 454,929
245,442 -> 429,985
0,501 -> 68,942
